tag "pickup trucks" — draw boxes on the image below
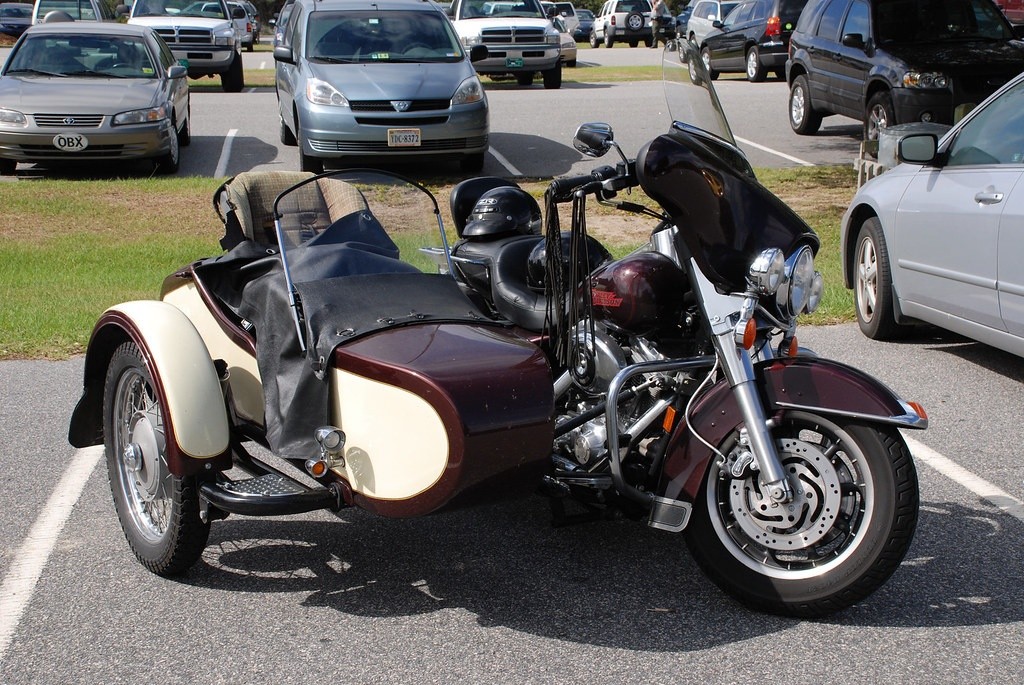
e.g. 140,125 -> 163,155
785,0 -> 1024,136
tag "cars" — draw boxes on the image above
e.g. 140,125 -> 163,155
570,9 -> 596,42
0,3 -> 34,36
676,0 -> 698,38
540,1 -> 580,68
0,21 -> 191,176
839,71 -> 1024,361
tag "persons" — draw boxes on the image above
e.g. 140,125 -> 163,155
649,0 -> 668,47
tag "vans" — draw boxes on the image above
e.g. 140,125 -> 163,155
685,0 -> 741,48
32,0 -> 113,29
273,0 -> 491,176
699,0 -> 809,84
201,1 -> 255,52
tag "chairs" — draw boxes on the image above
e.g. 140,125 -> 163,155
229,170 -> 368,267
36,46 -> 78,73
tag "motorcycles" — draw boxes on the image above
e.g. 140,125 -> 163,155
69,37 -> 932,625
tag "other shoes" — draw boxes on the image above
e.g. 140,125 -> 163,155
650,46 -> 657,49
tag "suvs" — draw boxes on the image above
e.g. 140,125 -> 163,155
181,0 -> 260,42
589,0 -> 653,48
116,0 -> 244,92
656,3 -> 677,40
444,0 -> 563,89
268,0 -> 295,50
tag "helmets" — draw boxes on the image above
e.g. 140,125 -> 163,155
461,185 -> 542,238
